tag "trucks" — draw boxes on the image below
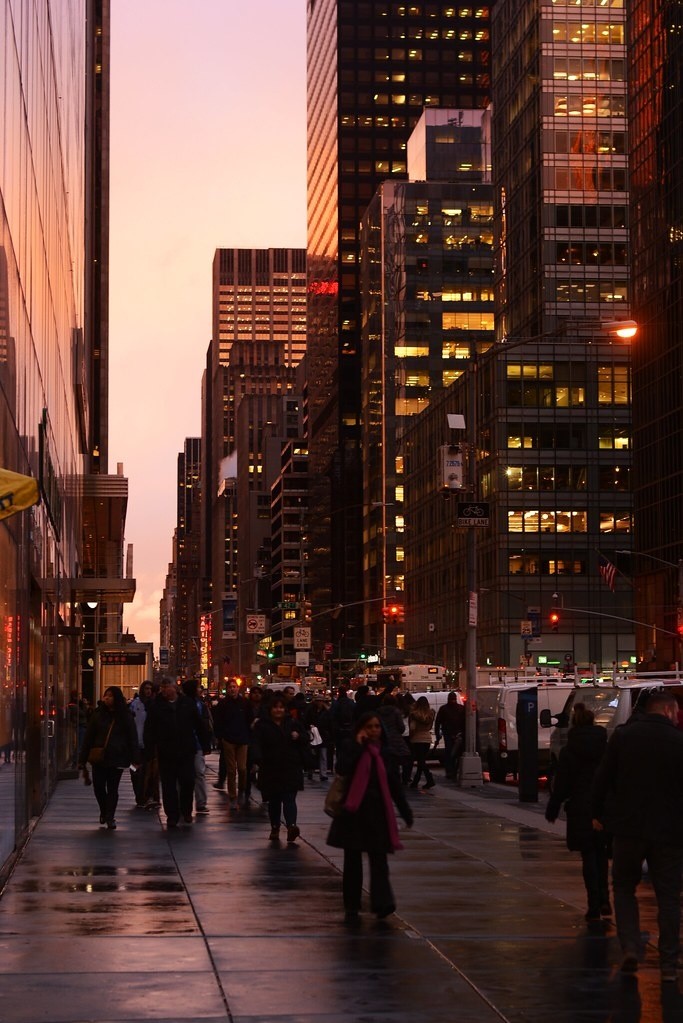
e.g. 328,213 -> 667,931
302,677 -> 327,698
459,666 -> 562,691
351,675 -> 378,691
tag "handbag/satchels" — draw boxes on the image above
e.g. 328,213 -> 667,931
324,773 -> 347,818
309,726 -> 322,745
87,747 -> 105,764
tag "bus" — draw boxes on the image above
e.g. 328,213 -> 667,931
377,664 -> 447,691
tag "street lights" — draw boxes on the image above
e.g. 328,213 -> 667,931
252,571 -> 300,688
180,617 -> 215,685
459,317 -> 638,789
614,549 -> 682,664
621,662 -> 629,679
480,587 -> 528,666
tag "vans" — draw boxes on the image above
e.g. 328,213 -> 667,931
540,664 -> 683,796
474,672 -> 584,782
261,683 -> 299,695
401,692 -> 465,765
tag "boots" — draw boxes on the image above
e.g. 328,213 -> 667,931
422,773 -> 435,789
407,774 -> 421,789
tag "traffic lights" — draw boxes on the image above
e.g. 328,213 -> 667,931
552,613 -> 559,633
486,657 -> 496,666
382,603 -> 405,625
361,650 -> 366,659
265,647 -> 274,660
302,601 -> 313,623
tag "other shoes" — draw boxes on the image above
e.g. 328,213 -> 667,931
619,956 -> 639,973
286,825 -> 299,841
307,773 -> 314,780
76,771 -> 266,831
660,963 -> 679,981
378,903 -> 396,920
585,907 -> 600,919
400,782 -> 407,790
320,776 -> 328,781
444,772 -> 457,778
344,910 -> 362,929
600,898 -> 613,915
269,825 -> 279,839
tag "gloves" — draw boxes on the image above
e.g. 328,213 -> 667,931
400,809 -> 413,829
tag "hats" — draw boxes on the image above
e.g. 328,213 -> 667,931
448,692 -> 456,700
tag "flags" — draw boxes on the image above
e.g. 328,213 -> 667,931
599,557 -> 617,594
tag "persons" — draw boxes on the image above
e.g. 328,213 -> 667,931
336,711 -> 412,928
544,693 -> 683,982
70,676 -> 479,842
